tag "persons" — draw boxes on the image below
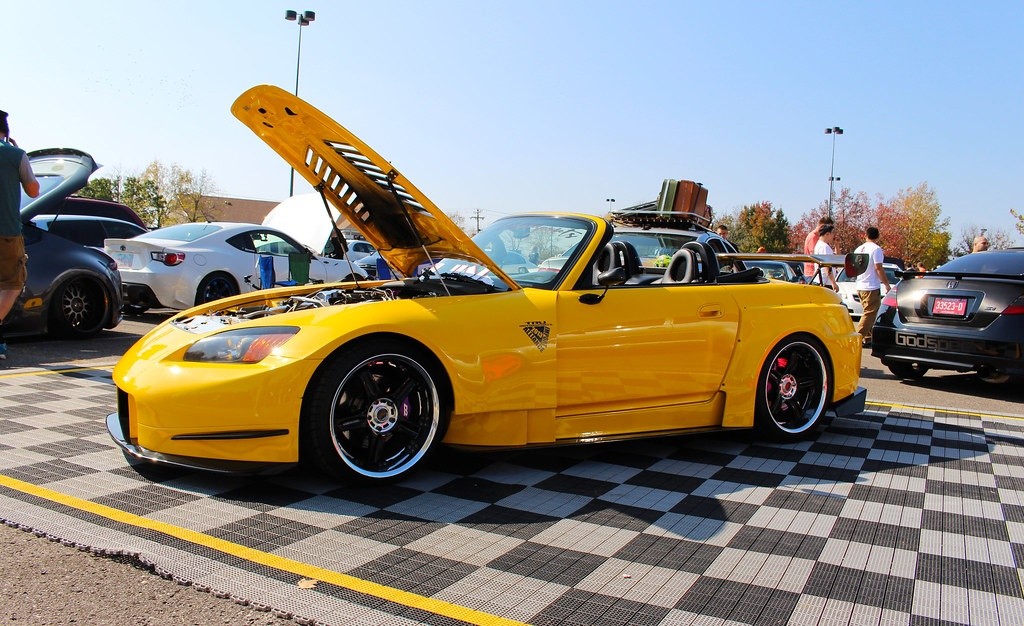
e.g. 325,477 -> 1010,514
491,236 -> 507,268
717,225 -> 740,253
907,262 -> 926,279
0,109 -> 39,360
329,230 -> 349,260
804,216 -> 841,293
854,227 -> 891,348
528,246 -> 539,264
972,236 -> 987,253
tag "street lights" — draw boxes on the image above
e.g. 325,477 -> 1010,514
284,9 -> 316,197
824,126 -> 844,218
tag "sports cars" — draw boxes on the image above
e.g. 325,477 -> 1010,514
105,83 -> 867,488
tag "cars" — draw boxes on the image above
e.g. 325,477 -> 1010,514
835,263 -> 903,318
496,221 -> 803,286
104,191 -> 377,315
871,246 -> 1024,387
0,147 -> 150,342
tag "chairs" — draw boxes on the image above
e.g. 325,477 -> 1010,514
647,240 -> 721,284
592,242 -> 631,287
885,273 -> 896,283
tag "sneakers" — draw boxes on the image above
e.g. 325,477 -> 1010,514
0,342 -> 8,360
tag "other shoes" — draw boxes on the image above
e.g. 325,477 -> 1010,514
860,340 -> 872,349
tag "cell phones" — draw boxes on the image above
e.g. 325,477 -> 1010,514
6,131 -> 9,143
